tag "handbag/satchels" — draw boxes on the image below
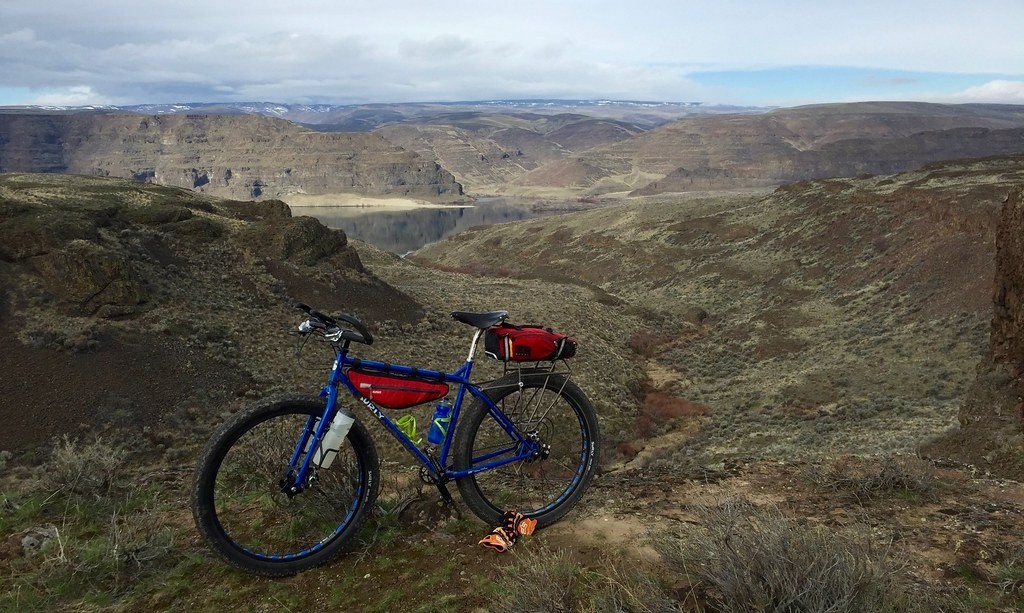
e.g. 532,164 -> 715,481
486,321 -> 578,362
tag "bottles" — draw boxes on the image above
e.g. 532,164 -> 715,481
428,397 -> 453,445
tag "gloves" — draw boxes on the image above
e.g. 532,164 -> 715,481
477,526 -> 517,552
499,511 -> 537,536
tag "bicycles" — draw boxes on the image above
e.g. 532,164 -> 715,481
192,304 -> 601,579
